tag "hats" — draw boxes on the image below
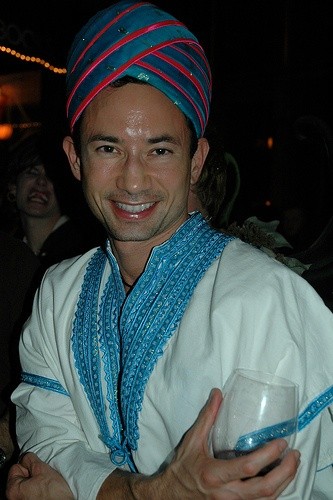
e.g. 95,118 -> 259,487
65,1 -> 211,139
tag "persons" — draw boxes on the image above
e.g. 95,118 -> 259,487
6,0 -> 333,500
0,130 -> 333,403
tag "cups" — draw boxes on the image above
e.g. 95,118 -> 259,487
212,368 -> 298,482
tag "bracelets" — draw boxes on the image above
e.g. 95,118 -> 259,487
0,448 -> 6,464
